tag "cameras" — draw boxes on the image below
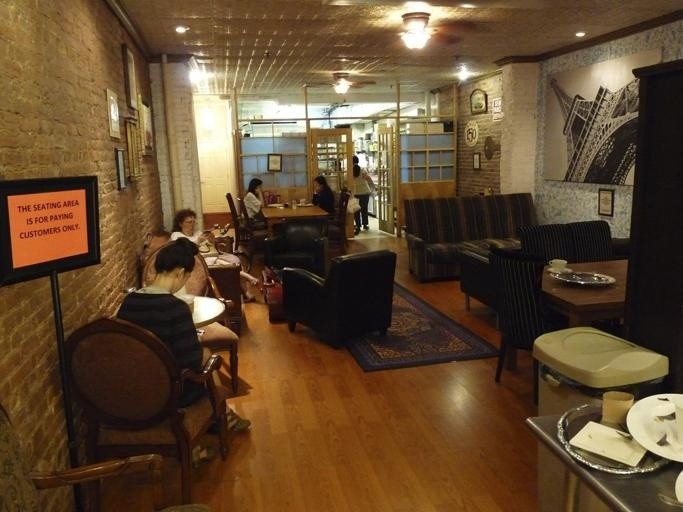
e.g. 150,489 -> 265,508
195,328 -> 204,336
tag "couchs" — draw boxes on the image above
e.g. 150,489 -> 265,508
491,217 -> 630,405
401,192 -> 536,282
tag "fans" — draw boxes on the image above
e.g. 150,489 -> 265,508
334,13 -> 484,34
299,72 -> 377,90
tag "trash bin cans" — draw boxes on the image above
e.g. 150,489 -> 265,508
531,327 -> 670,512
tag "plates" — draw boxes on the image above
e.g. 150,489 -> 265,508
626,393 -> 682,463
547,267 -> 572,273
675,470 -> 683,503
567,419 -> 647,468
550,271 -> 617,286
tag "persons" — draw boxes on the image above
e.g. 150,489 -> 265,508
331,159 -> 341,171
311,174 -> 335,222
169,208 -> 259,302
343,155 -> 374,230
240,179 -> 282,268
116,241 -> 251,433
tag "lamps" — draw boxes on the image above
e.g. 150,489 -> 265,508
334,83 -> 350,94
398,28 -> 431,51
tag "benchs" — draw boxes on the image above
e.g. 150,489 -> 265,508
455,246 -> 514,327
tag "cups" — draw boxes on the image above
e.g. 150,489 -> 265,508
300,199 -> 305,205
291,200 -> 296,209
549,259 -> 567,270
671,394 -> 682,443
602,391 -> 633,423
185,293 -> 194,316
276,195 -> 280,203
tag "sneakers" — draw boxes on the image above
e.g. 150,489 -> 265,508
355,225 -> 369,231
219,407 -> 252,434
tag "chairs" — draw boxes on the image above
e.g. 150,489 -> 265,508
0,405 -> 206,511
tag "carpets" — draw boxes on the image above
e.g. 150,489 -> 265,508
346,278 -> 499,374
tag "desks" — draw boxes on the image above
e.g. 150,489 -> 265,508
538,259 -> 626,325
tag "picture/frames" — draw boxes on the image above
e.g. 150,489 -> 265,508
473,152 -> 481,171
470,88 -> 487,114
108,37 -> 154,190
597,188 -> 614,217
267,153 -> 282,172
